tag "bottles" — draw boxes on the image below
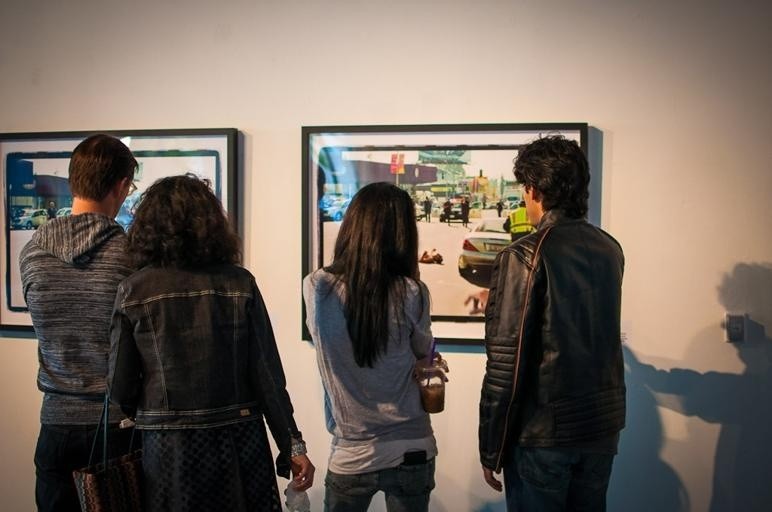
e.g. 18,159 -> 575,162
286,479 -> 310,511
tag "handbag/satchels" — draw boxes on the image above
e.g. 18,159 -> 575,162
72,395 -> 143,511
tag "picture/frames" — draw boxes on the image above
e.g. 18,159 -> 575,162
0,128 -> 239,331
302,124 -> 588,346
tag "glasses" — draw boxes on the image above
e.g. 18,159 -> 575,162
128,179 -> 137,196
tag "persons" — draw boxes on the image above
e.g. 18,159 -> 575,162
498,203 -> 503,218
302,181 -> 449,512
18,134 -> 139,512
464,133 -> 627,512
503,201 -> 534,241
424,196 -> 431,222
107,172 -> 314,512
443,202 -> 451,222
48,202 -> 57,219
461,197 -> 469,227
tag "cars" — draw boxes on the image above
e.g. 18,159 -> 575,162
323,199 -> 350,222
459,216 -> 513,277
440,202 -> 469,223
415,204 -> 427,220
12,207 -> 71,230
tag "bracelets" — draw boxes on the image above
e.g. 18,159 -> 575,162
290,442 -> 307,457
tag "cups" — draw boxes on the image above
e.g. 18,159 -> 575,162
415,359 -> 447,413
